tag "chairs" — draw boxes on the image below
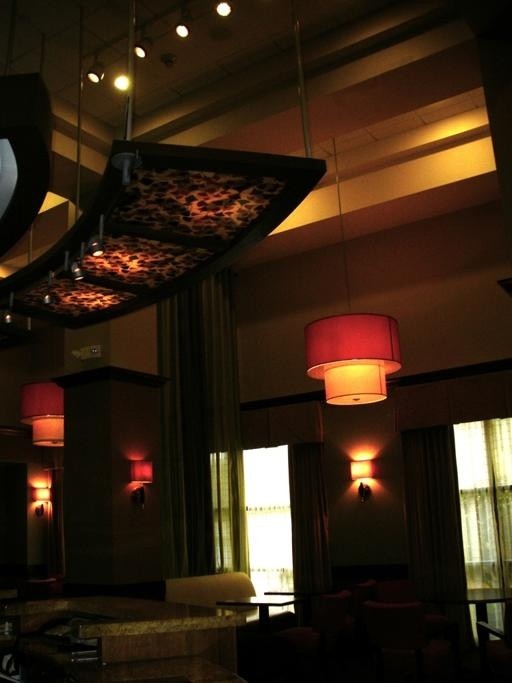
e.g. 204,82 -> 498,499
273,579 -> 510,681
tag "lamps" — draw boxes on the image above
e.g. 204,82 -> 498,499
127,456 -> 153,485
299,9 -> 405,405
83,2 -> 232,84
17,382 -> 68,450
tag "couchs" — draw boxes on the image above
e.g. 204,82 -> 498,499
161,568 -> 256,647
